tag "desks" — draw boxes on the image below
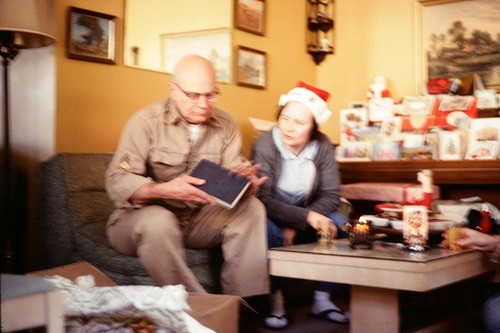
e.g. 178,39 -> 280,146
267,239 -> 491,333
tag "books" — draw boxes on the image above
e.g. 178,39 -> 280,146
189,158 -> 250,209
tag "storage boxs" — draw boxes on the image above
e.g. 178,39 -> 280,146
28,260 -> 257,333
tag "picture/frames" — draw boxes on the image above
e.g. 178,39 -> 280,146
65,5 -> 117,64
237,44 -> 268,89
235,0 -> 266,36
416,0 -> 500,93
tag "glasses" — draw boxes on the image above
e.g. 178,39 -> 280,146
174,82 -> 220,101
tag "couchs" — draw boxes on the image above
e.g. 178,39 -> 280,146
37,154 -> 226,292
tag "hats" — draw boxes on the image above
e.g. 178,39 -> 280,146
278,82 -> 331,126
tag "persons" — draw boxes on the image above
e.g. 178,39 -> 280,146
365,74 -> 395,106
102,55 -> 270,298
440,228 -> 500,333
250,81 -> 349,328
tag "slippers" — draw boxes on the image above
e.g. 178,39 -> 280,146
265,313 -> 287,329
311,306 -> 349,325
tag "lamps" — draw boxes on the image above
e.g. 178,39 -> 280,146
0,0 -> 59,273
